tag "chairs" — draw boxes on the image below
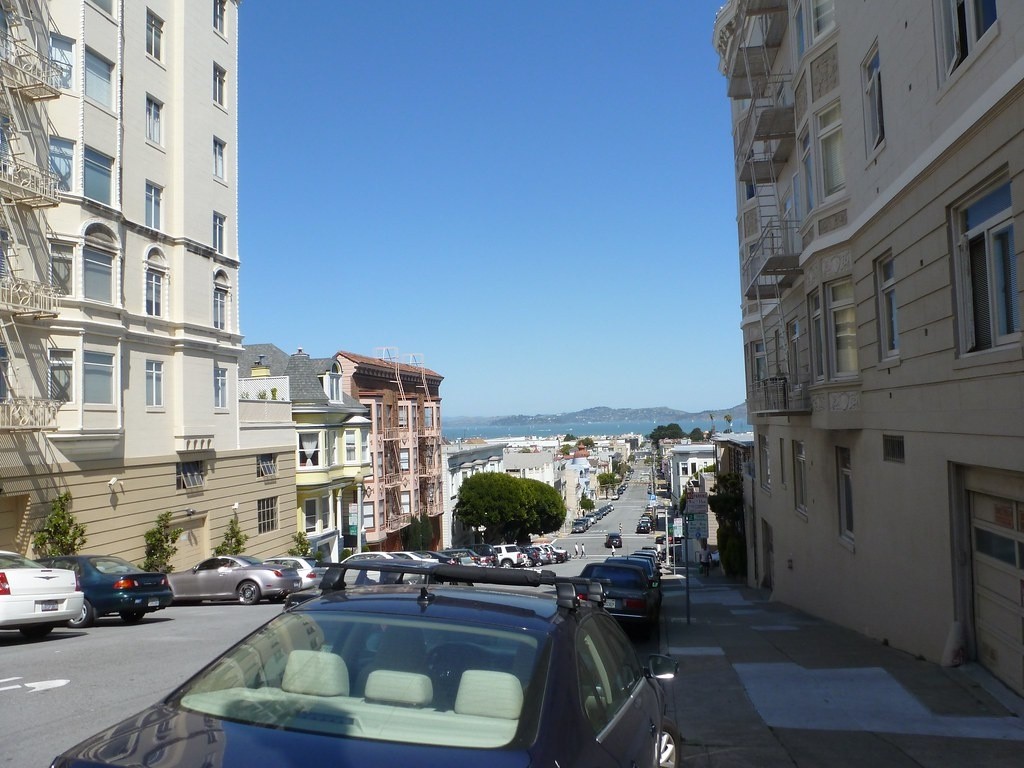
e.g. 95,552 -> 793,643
285,651 -> 524,720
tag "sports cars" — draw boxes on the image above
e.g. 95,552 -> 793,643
164,555 -> 303,608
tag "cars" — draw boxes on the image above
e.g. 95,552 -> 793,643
636,505 -> 654,533
611,483 -> 628,500
710,549 -> 720,566
625,469 -> 634,481
571,503 -> 614,533
655,533 -> 680,545
605,533 -> 623,548
0,549 -> 85,640
572,546 -> 661,638
263,556 -> 328,591
32,554 -> 174,628
388,551 -> 440,567
416,550 -> 461,566
313,552 -> 425,590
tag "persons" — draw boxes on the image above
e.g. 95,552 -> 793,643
619,522 -> 622,536
572,542 -> 588,559
610,543 -> 615,557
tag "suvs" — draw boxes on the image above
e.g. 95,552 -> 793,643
519,542 -> 571,568
47,562 -> 680,768
439,549 -> 481,567
452,544 -> 500,567
488,546 -> 524,569
